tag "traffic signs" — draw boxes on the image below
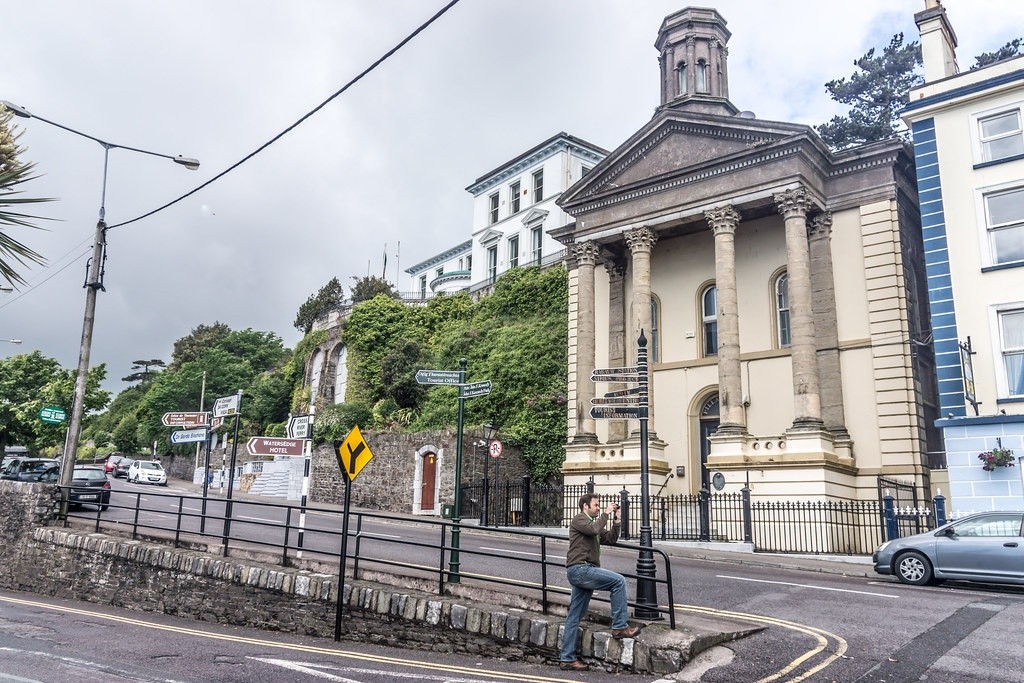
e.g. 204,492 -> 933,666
285,415 -> 308,439
162,411 -> 224,444
590,368 -> 639,419
212,396 -> 237,416
247,437 -> 305,458
415,370 -> 459,385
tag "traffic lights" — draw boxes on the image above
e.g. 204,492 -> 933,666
463,380 -> 492,398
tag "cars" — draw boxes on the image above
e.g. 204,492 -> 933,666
113,458 -> 135,477
126,459 -> 167,486
872,510 -> 1024,589
34,465 -> 111,511
104,455 -> 125,473
1,456 -> 59,483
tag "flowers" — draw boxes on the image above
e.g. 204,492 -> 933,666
977,447 -> 1015,471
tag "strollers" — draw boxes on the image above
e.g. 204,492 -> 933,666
201,473 -> 213,489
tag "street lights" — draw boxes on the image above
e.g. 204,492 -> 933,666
0,99 -> 199,520
482,423 -> 497,526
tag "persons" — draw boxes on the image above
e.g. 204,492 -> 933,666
559,494 -> 640,671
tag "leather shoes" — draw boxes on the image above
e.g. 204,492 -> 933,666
559,660 -> 589,671
612,627 -> 640,639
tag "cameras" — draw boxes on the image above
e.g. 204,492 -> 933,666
613,504 -> 620,510
